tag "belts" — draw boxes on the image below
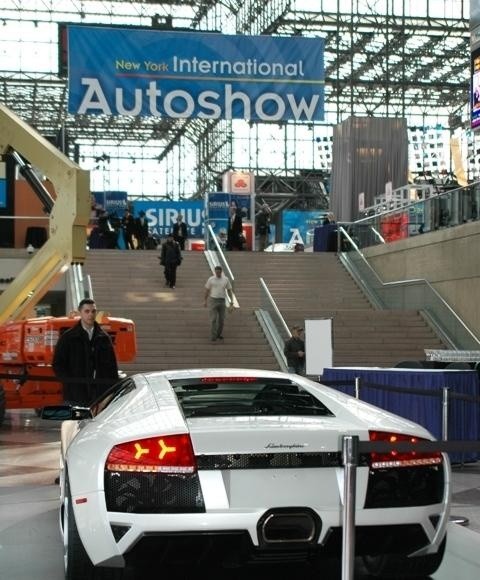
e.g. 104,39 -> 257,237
211,297 -> 225,301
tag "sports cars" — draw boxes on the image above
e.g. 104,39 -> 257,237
34,366 -> 455,578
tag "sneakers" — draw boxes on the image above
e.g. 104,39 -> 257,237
217,335 -> 224,339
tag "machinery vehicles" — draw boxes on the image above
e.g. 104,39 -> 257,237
0,100 -> 138,424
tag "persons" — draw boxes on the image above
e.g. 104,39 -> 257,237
226,207 -> 243,251
171,214 -> 188,251
159,232 -> 184,289
254,203 -> 271,253
322,211 -> 335,226
283,324 -> 307,378
52,298 -> 119,485
98,207 -> 117,240
285,166 -> 287,168
88,192 -> 105,217
202,264 -> 234,341
133,210 -> 149,250
228,198 -> 237,228
120,208 -> 136,249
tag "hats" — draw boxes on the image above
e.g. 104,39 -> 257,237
293,324 -> 304,331
